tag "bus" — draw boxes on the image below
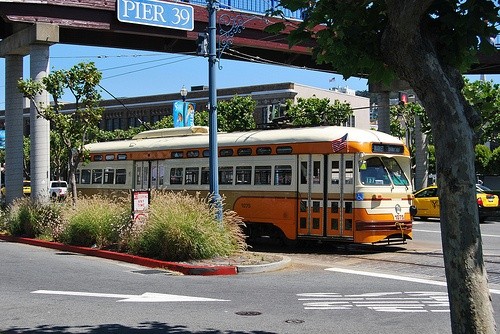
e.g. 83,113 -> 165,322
70,84 -> 417,255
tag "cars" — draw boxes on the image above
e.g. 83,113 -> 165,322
412,183 -> 500,223
0,180 -> 31,198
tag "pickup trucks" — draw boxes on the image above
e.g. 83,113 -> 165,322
49,181 -> 70,202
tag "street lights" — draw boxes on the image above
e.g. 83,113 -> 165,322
179,85 -> 189,127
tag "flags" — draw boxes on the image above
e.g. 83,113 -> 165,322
331,133 -> 347,152
